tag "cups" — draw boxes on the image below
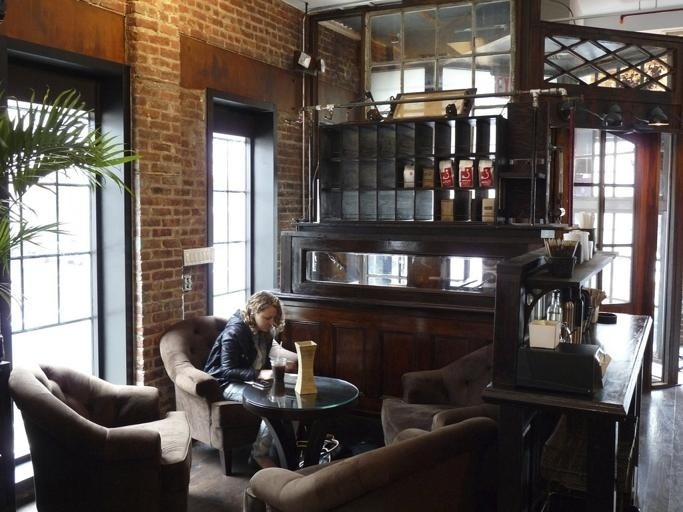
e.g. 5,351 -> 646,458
267,358 -> 287,382
545,255 -> 578,279
563,230 -> 589,263
269,382 -> 285,402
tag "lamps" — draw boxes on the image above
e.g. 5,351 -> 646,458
633,106 -> 670,127
556,100 -> 624,133
310,58 -> 326,73
294,50 -> 312,70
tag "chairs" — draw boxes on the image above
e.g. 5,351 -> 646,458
381,344 -> 522,466
9,359 -> 195,509
160,318 -> 298,476
242,416 -> 497,510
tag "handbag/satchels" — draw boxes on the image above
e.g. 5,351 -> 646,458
295,434 -> 339,467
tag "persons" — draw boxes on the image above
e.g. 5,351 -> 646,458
203,291 -> 286,470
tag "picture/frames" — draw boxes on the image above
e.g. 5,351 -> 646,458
386,87 -> 477,117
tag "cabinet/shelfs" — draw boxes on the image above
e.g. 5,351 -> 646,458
316,114 -> 508,224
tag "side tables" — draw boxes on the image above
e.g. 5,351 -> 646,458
223,375 -> 361,467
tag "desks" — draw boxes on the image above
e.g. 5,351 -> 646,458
480,310 -> 653,512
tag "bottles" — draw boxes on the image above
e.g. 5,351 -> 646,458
546,289 -> 562,342
